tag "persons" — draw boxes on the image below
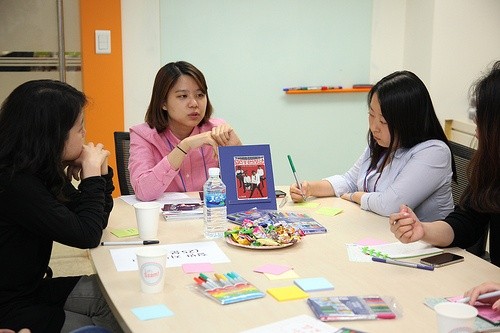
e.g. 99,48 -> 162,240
389,59 -> 500,266
248,170 -> 265,198
464,282 -> 500,311
290,70 -> 458,222
244,173 -> 251,191
257,166 -> 265,187
0,80 -> 115,333
236,166 -> 246,193
128,62 -> 242,202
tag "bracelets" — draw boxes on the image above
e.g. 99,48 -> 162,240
176,145 -> 187,154
349,192 -> 354,201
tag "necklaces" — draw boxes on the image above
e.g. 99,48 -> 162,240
363,169 -> 381,192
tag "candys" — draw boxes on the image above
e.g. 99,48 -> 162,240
225,219 -> 306,246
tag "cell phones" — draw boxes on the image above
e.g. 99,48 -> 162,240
420,253 -> 464,267
164,204 -> 199,211
275,189 -> 286,197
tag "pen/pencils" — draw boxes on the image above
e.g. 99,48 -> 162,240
309,295 -> 397,323
372,256 -> 434,271
287,155 -> 306,201
194,271 -> 264,302
393,205 -> 410,225
457,290 -> 500,304
101,241 -> 159,246
283,86 -> 344,91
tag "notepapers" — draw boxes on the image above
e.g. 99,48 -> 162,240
110,228 -> 139,238
295,278 -> 334,293
267,285 -> 309,302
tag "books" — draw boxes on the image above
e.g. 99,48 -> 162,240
161,203 -> 203,220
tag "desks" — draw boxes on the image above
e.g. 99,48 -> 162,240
88,185 -> 499,333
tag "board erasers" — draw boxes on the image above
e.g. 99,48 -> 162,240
353,84 -> 373,89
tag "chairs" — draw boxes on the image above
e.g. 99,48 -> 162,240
447,139 -> 490,261
114,131 -> 135,195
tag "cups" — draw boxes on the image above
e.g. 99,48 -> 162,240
434,302 -> 478,333
133,202 -> 161,240
135,248 -> 168,294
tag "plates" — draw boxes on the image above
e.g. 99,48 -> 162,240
224,236 -> 298,249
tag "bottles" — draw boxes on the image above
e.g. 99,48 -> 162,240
203,167 -> 227,240
